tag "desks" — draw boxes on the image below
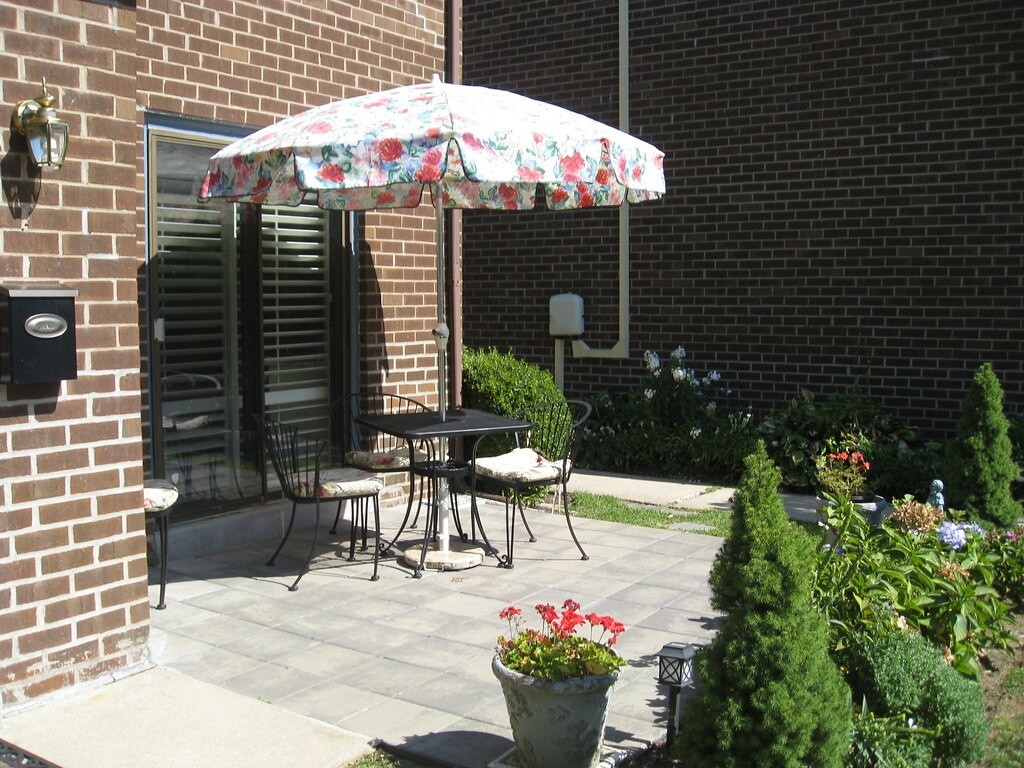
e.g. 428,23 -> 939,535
354,408 -> 533,577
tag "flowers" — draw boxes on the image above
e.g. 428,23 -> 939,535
494,599 -> 626,682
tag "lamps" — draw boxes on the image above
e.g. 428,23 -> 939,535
13,77 -> 69,170
655,642 -> 696,689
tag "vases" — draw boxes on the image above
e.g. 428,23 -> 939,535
492,646 -> 623,768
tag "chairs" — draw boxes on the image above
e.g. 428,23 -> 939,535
144,478 -> 179,610
466,399 -> 592,568
251,414 -> 384,591
161,374 -> 230,511
330,392 -> 432,550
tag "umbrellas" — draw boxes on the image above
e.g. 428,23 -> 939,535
202,76 -> 666,570
